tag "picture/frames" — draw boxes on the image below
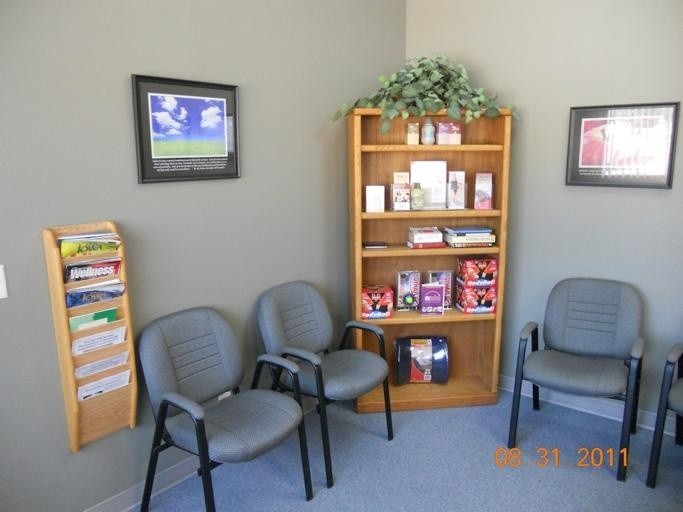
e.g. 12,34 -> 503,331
565,104 -> 680,189
131,73 -> 240,183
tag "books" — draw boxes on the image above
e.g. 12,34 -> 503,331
364,225 -> 497,249
410,160 -> 447,210
57,232 -> 131,400
396,270 -> 457,314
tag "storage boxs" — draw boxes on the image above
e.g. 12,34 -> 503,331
455,256 -> 498,314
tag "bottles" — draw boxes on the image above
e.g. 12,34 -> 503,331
420,117 -> 436,145
410,182 -> 425,210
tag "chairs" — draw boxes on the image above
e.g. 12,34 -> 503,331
253,281 -> 394,487
138,306 -> 317,511
507,279 -> 682,488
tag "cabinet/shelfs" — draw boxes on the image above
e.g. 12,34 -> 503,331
44,223 -> 141,452
347,104 -> 512,413
